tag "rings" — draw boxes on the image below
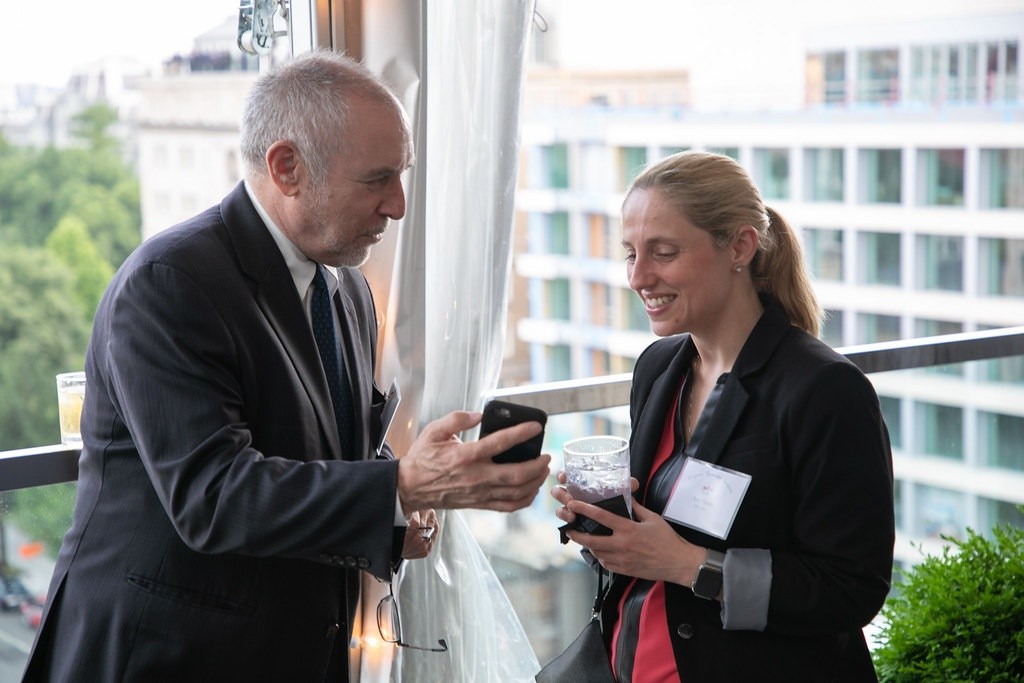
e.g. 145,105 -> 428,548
426,538 -> 431,542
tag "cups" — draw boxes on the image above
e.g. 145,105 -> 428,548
563,436 -> 632,520
56,371 -> 86,446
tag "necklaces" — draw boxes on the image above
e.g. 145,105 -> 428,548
688,355 -> 700,438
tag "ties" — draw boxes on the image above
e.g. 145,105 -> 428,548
309,263 -> 361,463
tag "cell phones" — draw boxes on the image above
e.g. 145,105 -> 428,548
477,401 -> 547,464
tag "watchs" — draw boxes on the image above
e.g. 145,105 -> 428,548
692,548 -> 724,601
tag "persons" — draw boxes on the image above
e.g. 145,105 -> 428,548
550,151 -> 895,683
21,51 -> 552,683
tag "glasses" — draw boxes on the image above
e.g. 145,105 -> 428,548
376,570 -> 449,655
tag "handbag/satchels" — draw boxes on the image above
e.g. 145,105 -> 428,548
534,563 -> 614,683
387,504 -> 542,683
